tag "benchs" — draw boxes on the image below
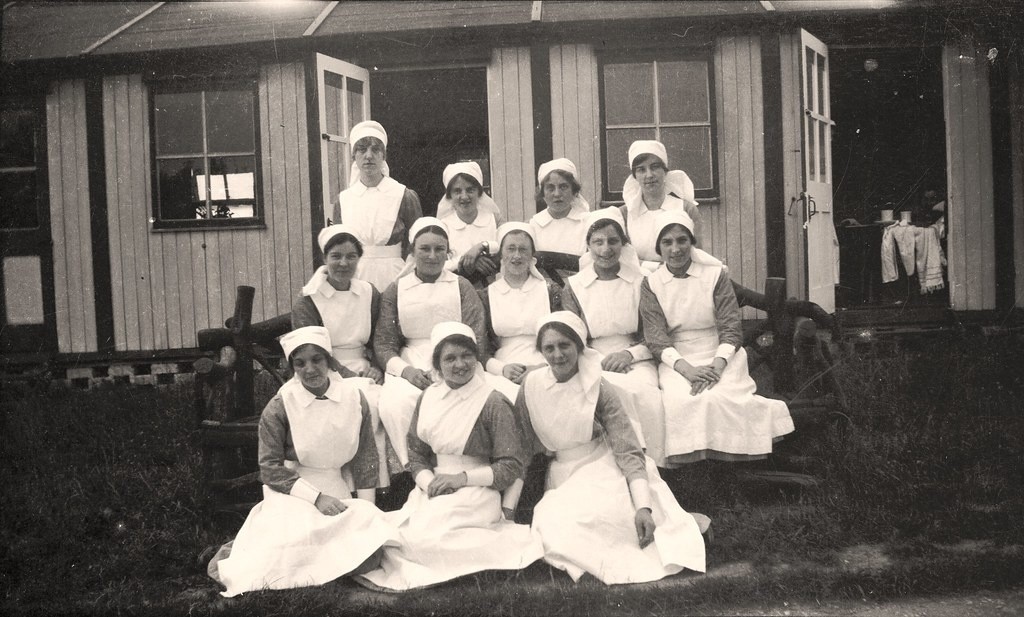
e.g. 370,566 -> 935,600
190,247 -> 844,478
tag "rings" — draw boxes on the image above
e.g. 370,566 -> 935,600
326,509 -> 330,513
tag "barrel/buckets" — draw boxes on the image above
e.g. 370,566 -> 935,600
881,210 -> 893,220
901,211 -> 912,222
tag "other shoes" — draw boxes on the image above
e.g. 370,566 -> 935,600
702,525 -> 714,548
196,543 -> 223,571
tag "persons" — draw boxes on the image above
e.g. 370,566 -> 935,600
502,310 -> 710,584
290,121 -> 795,489
405,321 -> 525,584
207,326 -> 384,598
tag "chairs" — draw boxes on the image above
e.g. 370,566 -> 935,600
845,223 -> 916,314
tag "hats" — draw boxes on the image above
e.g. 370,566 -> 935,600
408,216 -> 449,244
280,326 -> 332,362
350,120 -> 388,154
536,310 -> 588,348
443,162 -> 483,190
584,206 -> 626,245
431,322 -> 477,352
497,222 -> 536,252
653,210 -> 695,252
538,157 -> 578,189
318,224 -> 366,254
629,140 -> 668,170
